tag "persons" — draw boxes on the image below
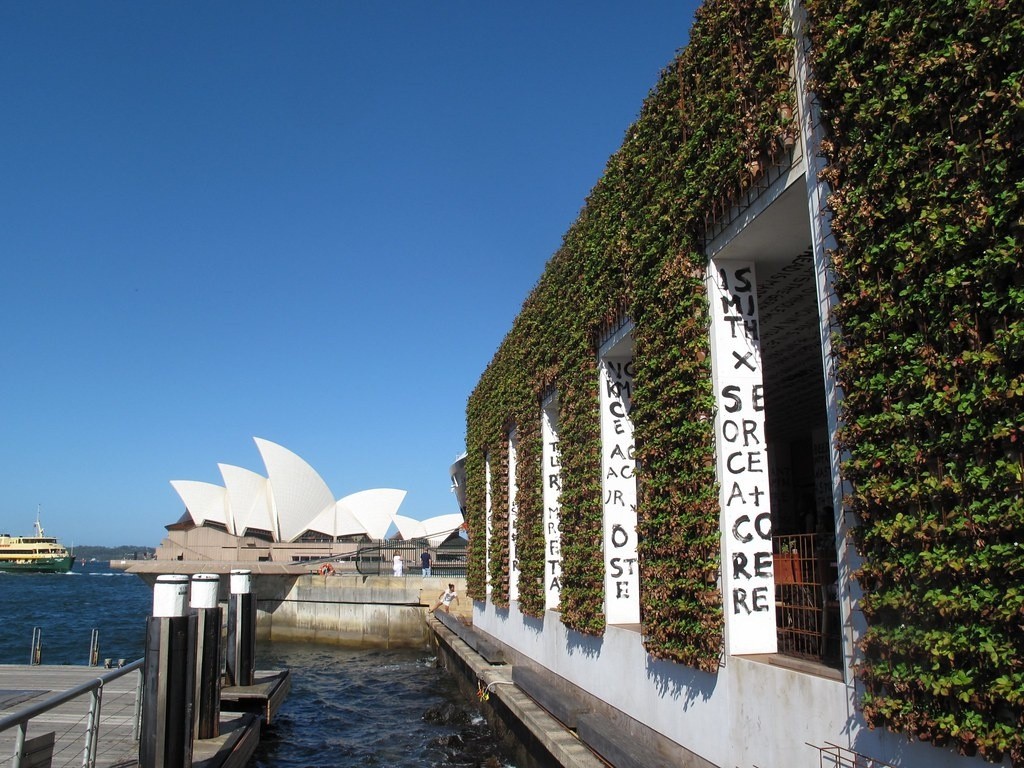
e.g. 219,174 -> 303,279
428,583 -> 459,614
813,505 -> 836,583
420,546 -> 432,577
393,551 -> 404,577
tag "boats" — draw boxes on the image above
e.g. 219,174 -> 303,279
0,503 -> 77,574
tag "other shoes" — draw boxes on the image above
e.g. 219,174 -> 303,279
428,609 -> 433,613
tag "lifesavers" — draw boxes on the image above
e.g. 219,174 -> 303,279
318,563 -> 335,577
